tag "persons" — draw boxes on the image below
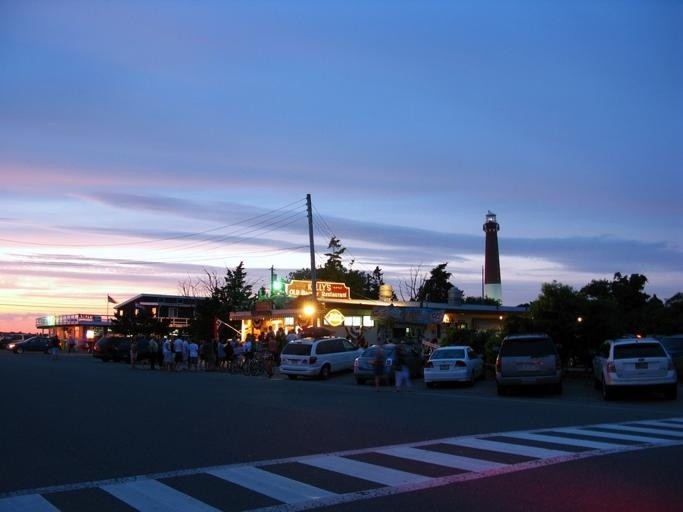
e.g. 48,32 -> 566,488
50,334 -> 61,359
66,335 -> 76,353
346,327 -> 369,348
370,328 -> 438,390
129,325 -> 319,374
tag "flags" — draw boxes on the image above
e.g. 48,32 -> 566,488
106,294 -> 118,304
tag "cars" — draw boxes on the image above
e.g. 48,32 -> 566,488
278,337 -> 482,386
93,336 -> 159,364
494,334 -> 563,394
0,334 -> 62,355
591,335 -> 678,396
